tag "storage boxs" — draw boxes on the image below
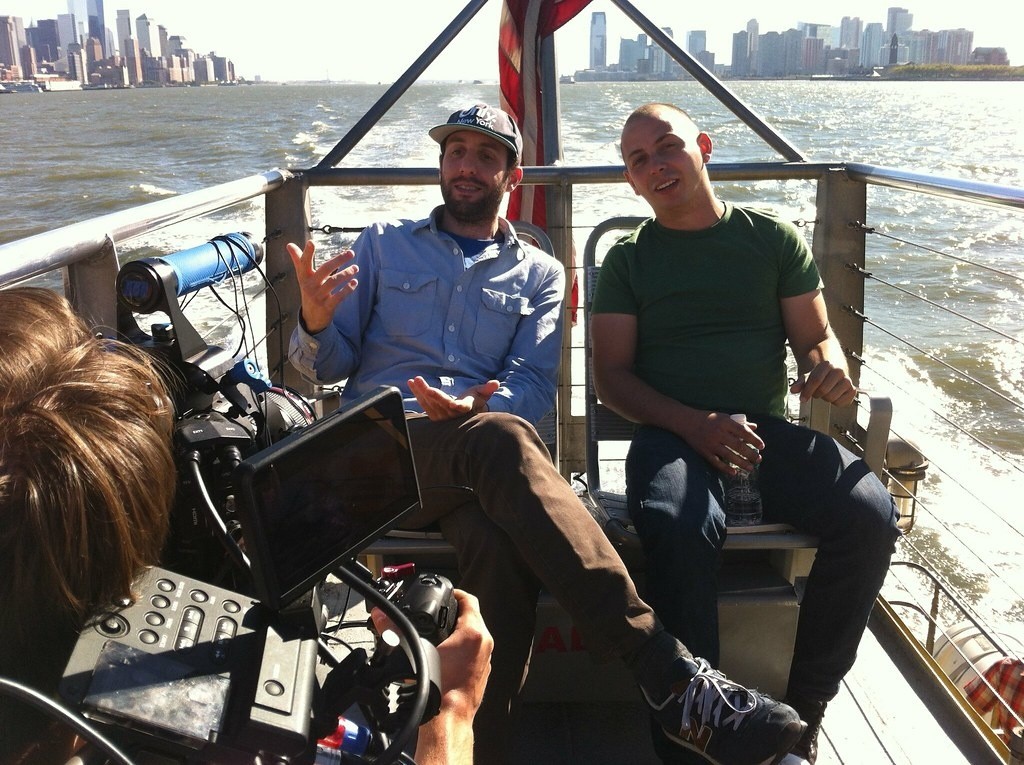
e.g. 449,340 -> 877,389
712,560 -> 800,698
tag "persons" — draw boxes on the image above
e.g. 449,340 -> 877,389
590,102 -> 899,765
0,286 -> 494,765
288,105 -> 807,765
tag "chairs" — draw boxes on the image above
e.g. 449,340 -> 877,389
361,221 -> 553,579
583,216 -> 821,552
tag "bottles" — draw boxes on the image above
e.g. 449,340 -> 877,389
721,414 -> 763,528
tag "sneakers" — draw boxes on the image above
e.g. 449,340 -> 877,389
778,683 -> 827,765
636,657 -> 808,765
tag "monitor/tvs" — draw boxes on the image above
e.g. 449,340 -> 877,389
232,384 -> 423,614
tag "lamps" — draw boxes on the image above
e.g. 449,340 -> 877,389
886,438 -> 929,535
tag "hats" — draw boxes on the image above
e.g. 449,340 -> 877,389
428,103 -> 523,164
